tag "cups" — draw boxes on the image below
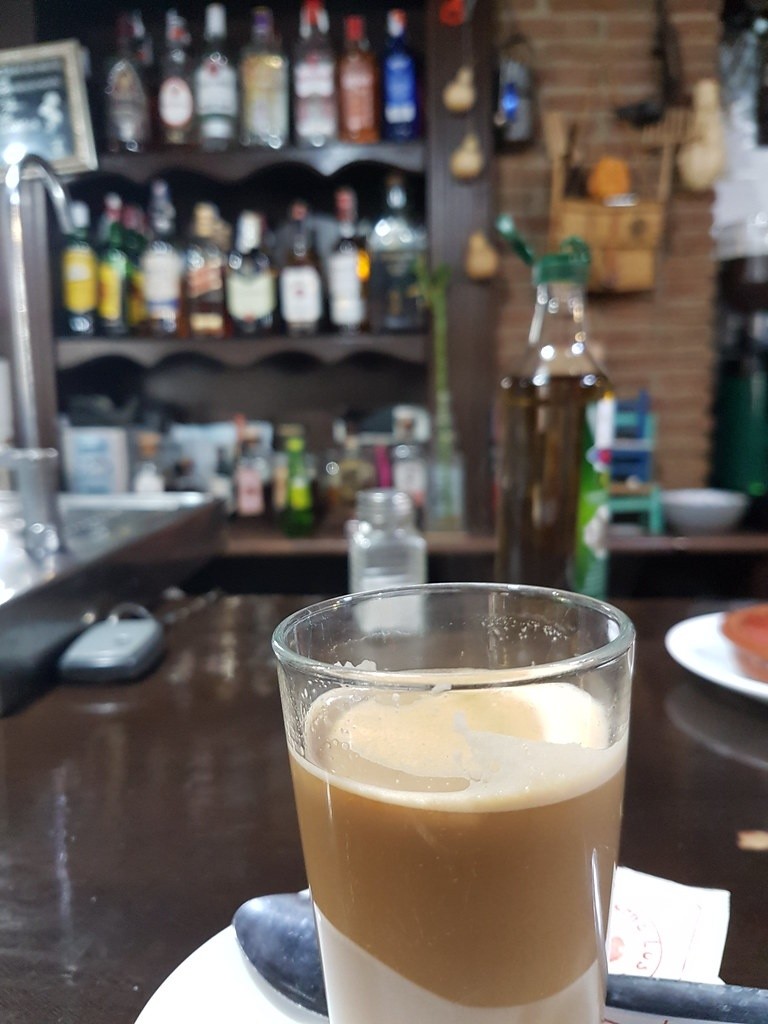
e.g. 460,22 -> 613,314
270,582 -> 635,1024
17,448 -> 58,556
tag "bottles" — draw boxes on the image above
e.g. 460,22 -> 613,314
327,190 -> 369,336
494,216 -> 618,602
240,5 -> 290,149
192,3 -> 239,148
62,202 -> 96,338
100,14 -> 153,152
347,489 -> 427,641
159,8 -> 193,149
366,176 -> 430,334
171,415 -> 426,535
95,178 -> 229,339
290,0 -> 337,148
277,206 -> 328,339
133,432 -> 168,496
337,19 -> 380,142
229,209 -> 277,339
382,11 -> 422,142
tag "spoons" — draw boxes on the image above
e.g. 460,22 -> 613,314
234,893 -> 768,1024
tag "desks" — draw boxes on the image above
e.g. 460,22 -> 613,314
0,585 -> 767,1024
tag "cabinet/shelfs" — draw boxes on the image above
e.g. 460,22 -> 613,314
42,0 -> 495,530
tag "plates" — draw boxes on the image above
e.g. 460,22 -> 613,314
135,889 -> 728,1024
666,678 -> 768,773
665,612 -> 768,701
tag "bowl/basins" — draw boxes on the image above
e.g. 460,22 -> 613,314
662,490 -> 746,536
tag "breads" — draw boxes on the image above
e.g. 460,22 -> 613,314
719,603 -> 768,684
